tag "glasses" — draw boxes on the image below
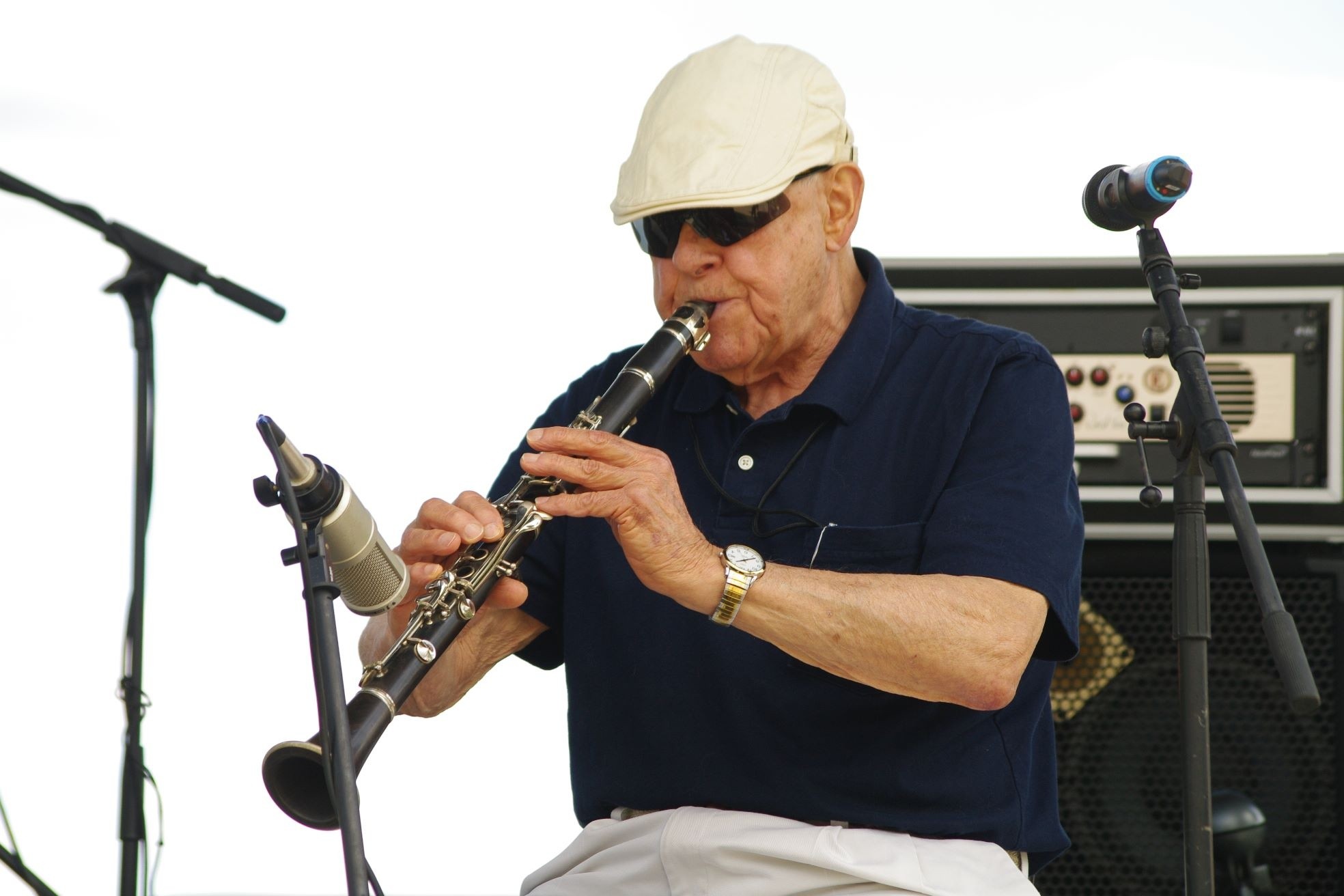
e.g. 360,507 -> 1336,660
632,164 -> 833,258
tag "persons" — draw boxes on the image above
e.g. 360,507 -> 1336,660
357,34 -> 1086,896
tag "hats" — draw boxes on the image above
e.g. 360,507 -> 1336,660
610,35 -> 858,225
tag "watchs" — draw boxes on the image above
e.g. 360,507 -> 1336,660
708,544 -> 765,627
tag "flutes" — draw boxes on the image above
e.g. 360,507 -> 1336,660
263,297 -> 715,832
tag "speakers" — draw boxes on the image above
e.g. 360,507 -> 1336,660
1030,527 -> 1344,895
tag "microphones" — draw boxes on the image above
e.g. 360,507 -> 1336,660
257,414 -> 412,616
1080,158 -> 1193,233
